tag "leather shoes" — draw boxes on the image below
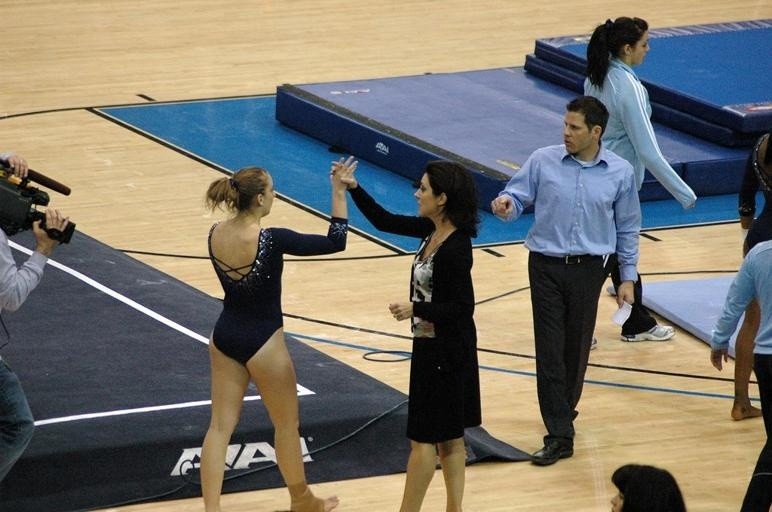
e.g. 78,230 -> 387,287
533,436 -> 573,470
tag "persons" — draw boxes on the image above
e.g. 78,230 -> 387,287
731,132 -> 772,421
585,16 -> 676,350
710,240 -> 772,512
612,466 -> 687,512
200,157 -> 358,512
0,152 -> 70,483
330,159 -> 482,512
491,96 -> 641,465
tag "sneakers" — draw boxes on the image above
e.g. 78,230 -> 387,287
590,337 -> 597,351
617,320 -> 679,342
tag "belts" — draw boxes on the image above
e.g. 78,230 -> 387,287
537,256 -> 605,265
